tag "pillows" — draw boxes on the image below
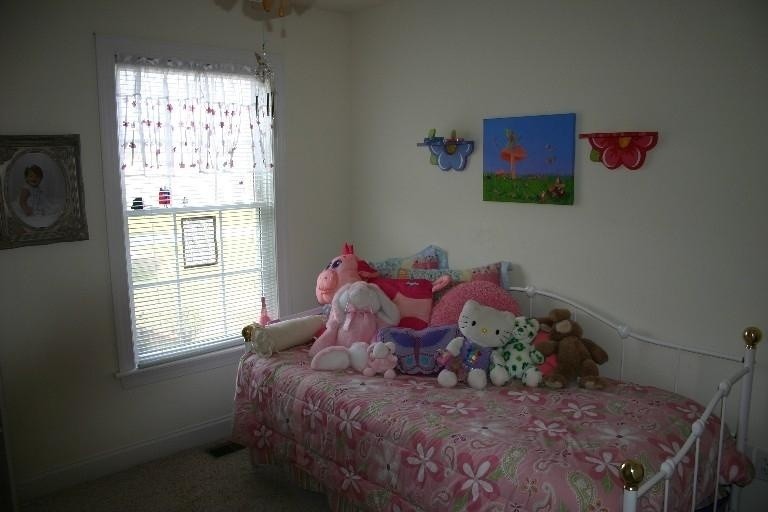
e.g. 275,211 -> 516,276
412,261 -> 511,297
369,245 -> 448,278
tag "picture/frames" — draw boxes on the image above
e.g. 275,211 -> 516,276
0,133 -> 89,251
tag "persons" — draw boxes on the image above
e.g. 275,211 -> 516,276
15,165 -> 60,216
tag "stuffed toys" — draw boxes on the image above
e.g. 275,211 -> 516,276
308,241 -> 608,389
309,242 -> 452,379
377,299 -> 608,390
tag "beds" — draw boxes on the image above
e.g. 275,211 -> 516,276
237,282 -> 762,512
